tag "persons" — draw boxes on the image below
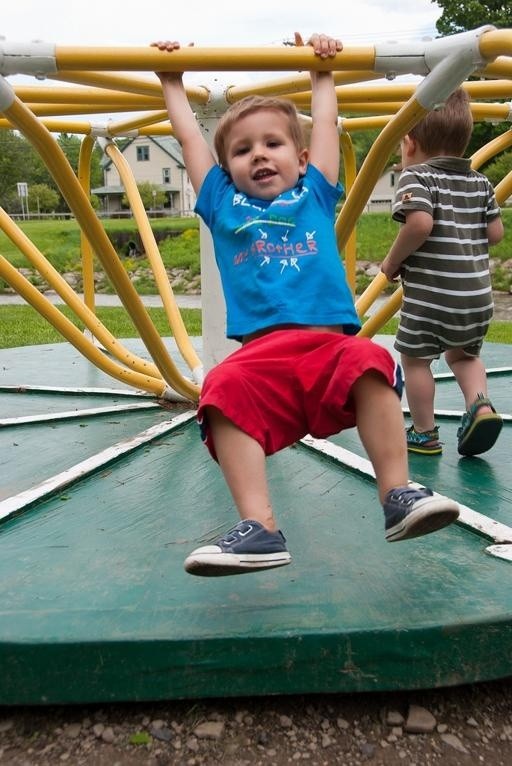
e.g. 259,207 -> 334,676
149,32 -> 455,578
381,84 -> 506,458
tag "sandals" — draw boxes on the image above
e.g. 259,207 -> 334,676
458,398 -> 504,455
406,424 -> 443,455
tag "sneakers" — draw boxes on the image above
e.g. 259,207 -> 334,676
379,487 -> 461,543
183,521 -> 292,578
